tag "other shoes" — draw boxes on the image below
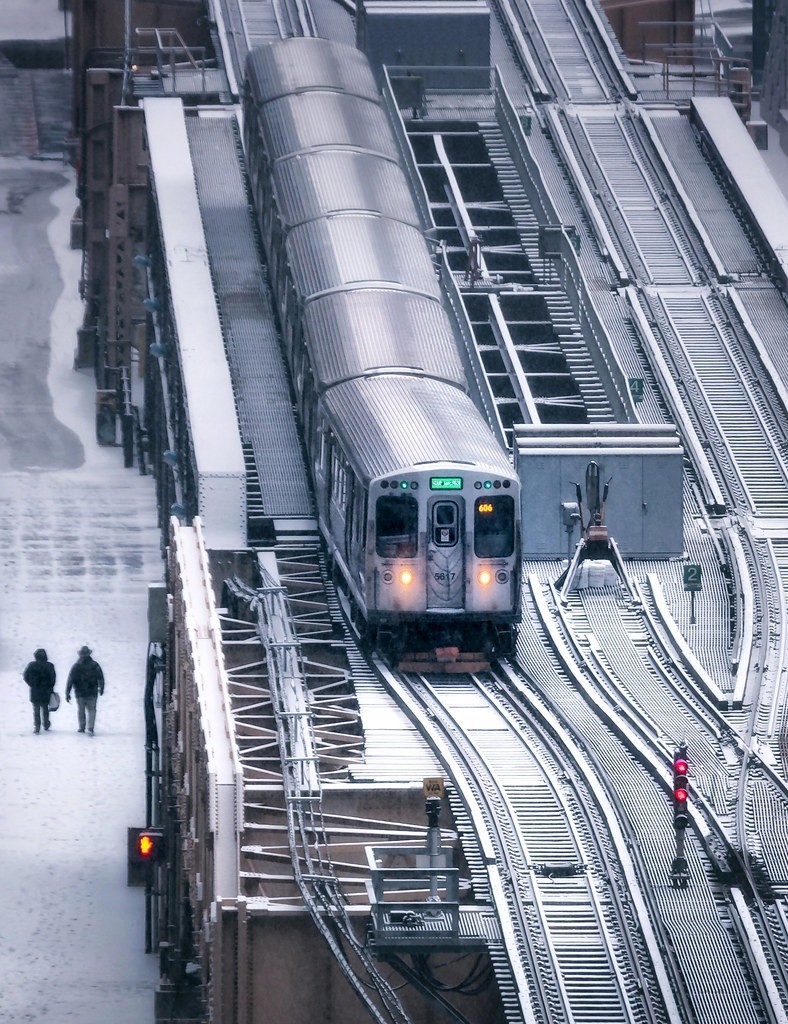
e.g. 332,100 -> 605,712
88,728 -> 93,735
44,721 -> 50,730
78,729 -> 84,732
33,730 -> 39,733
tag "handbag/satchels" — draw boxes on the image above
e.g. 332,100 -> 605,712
48,692 -> 60,711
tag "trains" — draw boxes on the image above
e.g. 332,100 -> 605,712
241,37 -> 525,672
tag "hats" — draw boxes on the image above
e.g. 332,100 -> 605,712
77,646 -> 92,657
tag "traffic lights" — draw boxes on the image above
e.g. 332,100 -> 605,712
673,748 -> 688,828
139,833 -> 163,859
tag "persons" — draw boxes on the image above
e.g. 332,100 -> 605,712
65,646 -> 106,737
23,649 -> 56,735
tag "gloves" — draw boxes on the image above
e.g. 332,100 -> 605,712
66,694 -> 71,703
99,688 -> 103,696
